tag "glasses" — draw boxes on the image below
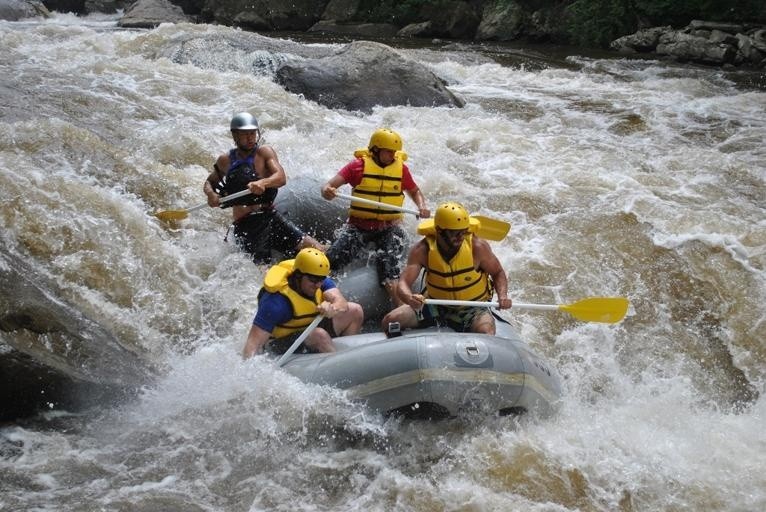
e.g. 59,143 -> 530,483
307,273 -> 326,282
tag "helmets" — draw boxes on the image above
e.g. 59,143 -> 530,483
368,128 -> 403,151
433,201 -> 470,230
292,247 -> 330,276
230,112 -> 259,131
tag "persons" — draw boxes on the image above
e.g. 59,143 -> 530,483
203,111 -> 326,264
242,248 -> 364,360
322,128 -> 430,307
382,201 -> 512,335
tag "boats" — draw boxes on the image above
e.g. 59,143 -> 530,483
270,172 -> 570,423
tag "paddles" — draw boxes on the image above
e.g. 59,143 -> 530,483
336,192 -> 511,242
155,188 -> 253,224
424,298 -> 628,323
